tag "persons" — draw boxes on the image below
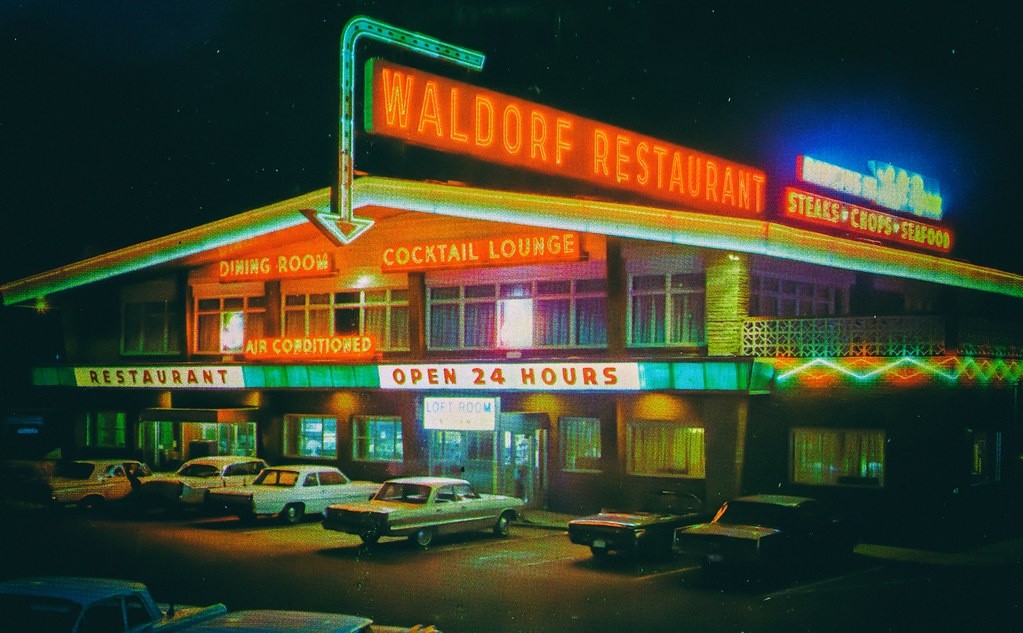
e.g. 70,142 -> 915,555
126,467 -> 141,491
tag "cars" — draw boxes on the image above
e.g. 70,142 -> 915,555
204,464 -> 394,526
568,489 -> 716,567
138,455 -> 315,518
322,475 -> 524,551
672,495 -> 854,582
28,458 -> 156,516
0,572 -> 442,633
